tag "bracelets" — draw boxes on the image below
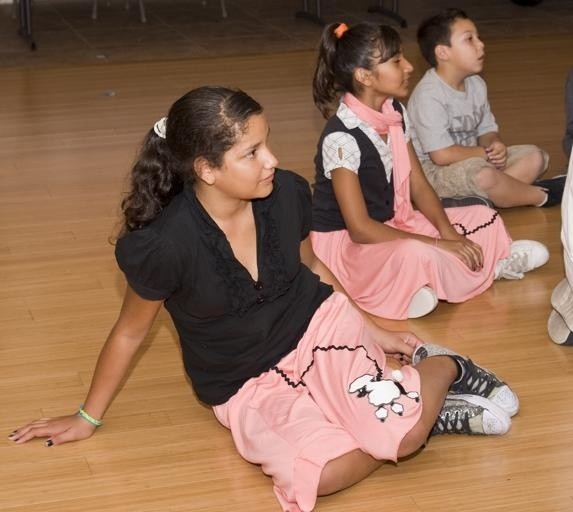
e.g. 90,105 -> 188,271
78,407 -> 103,426
435,236 -> 438,248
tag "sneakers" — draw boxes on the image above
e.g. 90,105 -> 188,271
408,285 -> 439,319
431,393 -> 513,436
412,343 -> 520,418
441,195 -> 495,208
488,239 -> 550,280
530,174 -> 567,208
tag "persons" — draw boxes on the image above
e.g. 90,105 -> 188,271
400,9 -> 567,212
306,18 -> 551,322
5,80 -> 524,512
